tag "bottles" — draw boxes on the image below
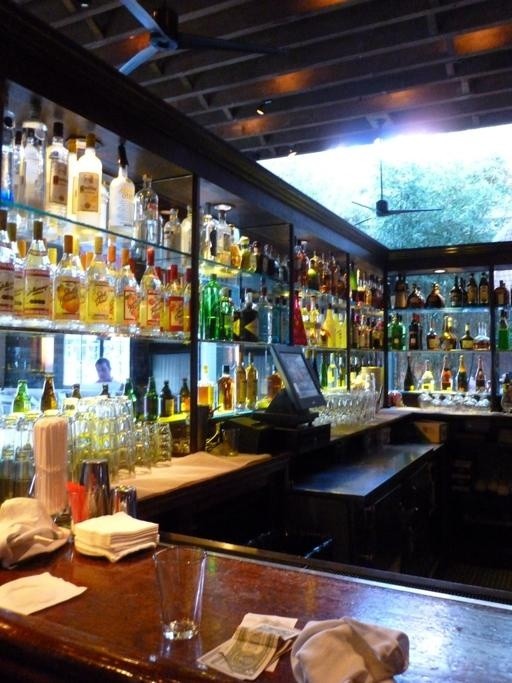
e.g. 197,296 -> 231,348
233,307 -> 240,342
8,222 -> 24,320
421,360 -> 434,392
475,356 -> 486,393
305,350 -> 311,369
115,247 -> 137,333
473,322 -> 491,350
198,365 -> 214,412
349,260 -> 385,305
214,203 -> 232,266
72,133 -> 102,240
272,297 -> 281,342
409,313 -> 419,349
495,280 -> 509,306
483,322 -> 491,350
253,241 -> 290,280
417,314 -> 423,349
109,145 -> 136,243
107,266 -> 115,327
505,311 -> 510,330
321,352 -> 327,387
0,109 -> 16,212
106,246 -> 120,276
220,287 -> 233,341
504,283 -> 506,289
72,239 -> 87,323
460,321 -> 474,349
217,365 -> 234,413
180,378 -> 190,411
48,247 -> 58,268
426,316 -> 439,349
140,248 -> 161,337
312,350 -> 318,380
40,373 -> 58,410
466,273 -> 477,305
22,220 -> 50,321
417,285 -> 420,296
266,364 -> 282,400
281,298 -> 289,343
155,265 -> 164,338
162,264 -> 183,339
182,205 -> 193,257
46,122 -> 69,228
295,241 -> 348,295
459,277 -> 467,306
479,272 -> 490,305
100,384 -> 110,397
239,287 -> 259,343
327,353 -> 338,387
440,355 -> 453,392
447,317 -> 457,349
498,310 -> 511,350
387,316 -> 393,350
202,274 -> 221,340
13,379 -> 31,413
163,270 -> 170,291
73,384 -> 80,399
183,267 -> 191,339
338,353 -> 345,387
123,378 -> 137,423
86,237 -> 109,333
202,214 -> 212,259
160,380 -> 174,417
350,354 -> 382,374
240,236 -> 257,272
236,351 -> 246,408
408,282 -> 426,307
456,355 -> 468,392
501,373 -> 512,412
450,275 -> 464,307
245,352 -> 259,410
350,308 -> 384,350
129,257 -> 141,326
425,283 -> 446,309
164,209 -> 181,260
404,356 -> 415,392
144,376 -> 158,422
397,314 -> 406,345
402,273 -> 408,289
394,273 -> 406,308
295,294 -> 348,348
258,287 -> 272,345
18,122 -> 48,220
391,314 -> 402,349
54,235 -> 81,323
134,175 -> 159,247
440,316 -> 453,351
0,210 -> 15,321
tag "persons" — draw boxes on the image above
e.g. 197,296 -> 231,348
95,357 -> 114,383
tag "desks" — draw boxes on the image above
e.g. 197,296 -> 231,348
382,405 -> 512,434
107,406 -> 411,517
1,498 -> 511,527
290,441 -> 445,553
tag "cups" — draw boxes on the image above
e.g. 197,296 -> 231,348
152,546 -> 207,640
90,486 -> 108,517
0,400 -> 173,482
65,486 -> 90,523
110,485 -> 138,520
315,389 -> 380,426
420,389 -> 492,413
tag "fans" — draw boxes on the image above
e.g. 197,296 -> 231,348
116,2 -> 282,78
348,112 -> 444,228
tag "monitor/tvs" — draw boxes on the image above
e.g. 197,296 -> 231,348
270,344 -> 327,411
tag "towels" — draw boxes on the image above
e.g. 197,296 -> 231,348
73,510 -> 159,563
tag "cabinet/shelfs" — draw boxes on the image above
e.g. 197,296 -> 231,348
0,2 -> 512,454
